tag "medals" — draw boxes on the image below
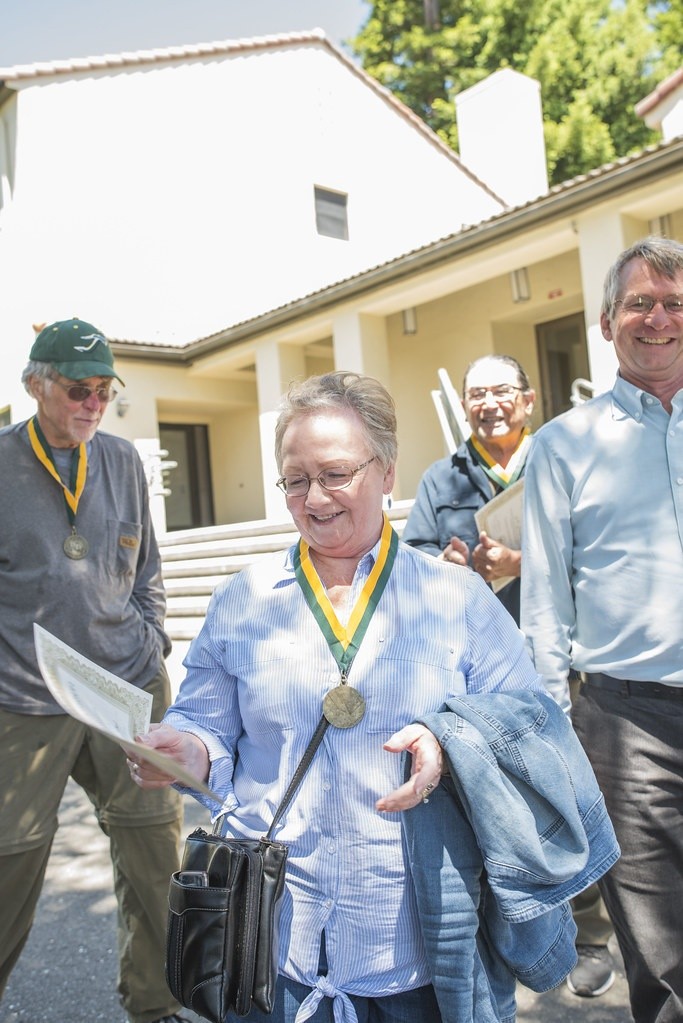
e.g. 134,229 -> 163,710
323,685 -> 366,728
63,530 -> 88,559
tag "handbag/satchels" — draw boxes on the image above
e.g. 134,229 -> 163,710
165,828 -> 290,1023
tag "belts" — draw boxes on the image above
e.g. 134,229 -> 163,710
577,667 -> 683,702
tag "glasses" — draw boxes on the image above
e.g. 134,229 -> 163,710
466,383 -> 526,405
277,456 -> 379,497
613,293 -> 683,316
46,373 -> 118,403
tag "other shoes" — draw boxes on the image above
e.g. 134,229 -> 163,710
153,1005 -> 212,1023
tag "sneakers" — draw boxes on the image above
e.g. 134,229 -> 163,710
565,943 -> 614,994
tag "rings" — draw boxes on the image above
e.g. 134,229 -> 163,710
133,764 -> 139,774
423,783 -> 435,804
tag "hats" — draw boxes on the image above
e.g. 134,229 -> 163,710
30,318 -> 124,389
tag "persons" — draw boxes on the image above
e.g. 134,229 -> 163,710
128,368 -> 540,1022
398,354 -> 616,1000
519,235 -> 683,1023
0,317 -> 211,1023
